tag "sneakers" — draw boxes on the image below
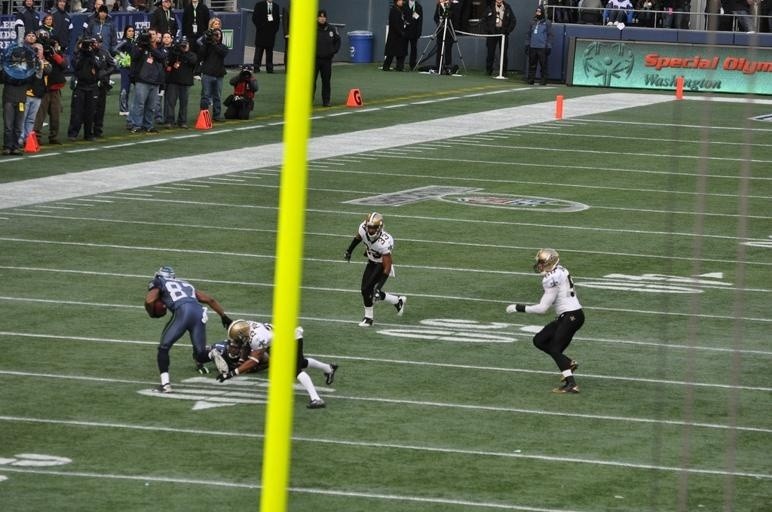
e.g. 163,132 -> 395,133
2,115 -> 225,157
324,363 -> 339,385
560,360 -> 579,382
307,399 -> 327,408
552,377 -> 580,394
149,384 -> 175,393
397,296 -> 406,318
359,318 -> 373,326
209,348 -> 229,375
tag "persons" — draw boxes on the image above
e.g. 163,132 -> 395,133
252,1 -> 282,71
311,8 -> 343,110
144,264 -> 233,393
191,335 -> 268,382
342,212 -> 407,327
378,1 -> 519,78
0,1 -> 259,155
216,317 -> 339,408
504,247 -> 585,394
547,0 -> 772,34
523,6 -> 552,86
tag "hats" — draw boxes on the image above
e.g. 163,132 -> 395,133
25,29 -> 36,35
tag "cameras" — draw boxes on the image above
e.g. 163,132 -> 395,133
240,67 -> 252,81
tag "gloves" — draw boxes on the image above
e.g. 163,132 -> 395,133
221,314 -> 233,329
196,364 -> 209,375
506,304 -> 525,315
343,250 -> 352,263
374,287 -> 381,301
215,370 -> 237,383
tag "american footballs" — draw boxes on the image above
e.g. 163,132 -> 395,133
153,300 -> 167,312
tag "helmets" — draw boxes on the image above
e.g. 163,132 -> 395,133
537,248 -> 560,273
152,266 -> 177,278
226,339 -> 241,358
363,212 -> 383,239
230,320 -> 250,343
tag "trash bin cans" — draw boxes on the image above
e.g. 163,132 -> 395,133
347,30 -> 374,63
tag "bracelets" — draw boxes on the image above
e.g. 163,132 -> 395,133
234,367 -> 240,376
378,273 -> 390,288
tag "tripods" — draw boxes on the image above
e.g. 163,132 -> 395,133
413,16 -> 470,77
